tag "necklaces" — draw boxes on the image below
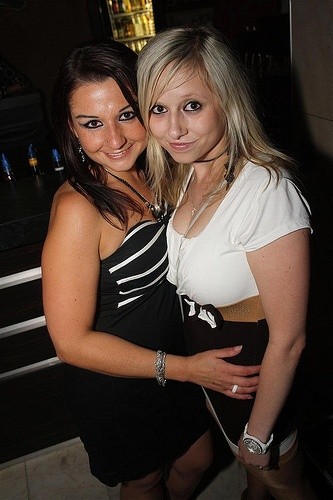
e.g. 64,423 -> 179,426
189,182 -> 225,217
103,168 -> 163,212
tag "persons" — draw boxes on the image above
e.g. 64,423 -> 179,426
138,27 -> 315,500
42,40 -> 264,500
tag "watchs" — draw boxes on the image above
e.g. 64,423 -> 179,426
243,422 -> 274,455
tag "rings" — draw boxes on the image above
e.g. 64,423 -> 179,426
257,465 -> 263,471
231,385 -> 239,394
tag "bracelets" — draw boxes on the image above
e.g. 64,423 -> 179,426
155,351 -> 167,387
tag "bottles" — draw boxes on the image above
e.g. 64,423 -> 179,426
50,144 -> 62,170
0,153 -> 16,184
28,145 -> 43,177
